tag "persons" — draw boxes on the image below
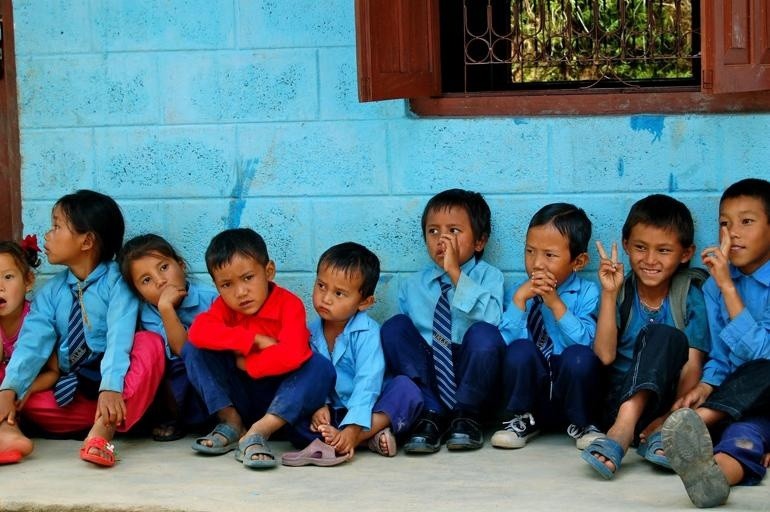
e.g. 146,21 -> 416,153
582,194 -> 770,480
661,178 -> 770,508
0,190 -> 166,467
0,235 -> 59,411
380,189 -> 505,452
491,204 -> 606,450
180,229 -> 337,469
120,234 -> 219,443
307,243 -> 423,457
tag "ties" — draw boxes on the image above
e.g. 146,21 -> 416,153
167,352 -> 189,398
433,277 -> 458,410
54,281 -> 96,408
527,295 -> 554,401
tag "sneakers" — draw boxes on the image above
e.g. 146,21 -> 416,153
567,424 -> 607,450
490,412 -> 541,448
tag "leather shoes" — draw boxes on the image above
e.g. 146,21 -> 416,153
405,419 -> 441,453
446,415 -> 484,449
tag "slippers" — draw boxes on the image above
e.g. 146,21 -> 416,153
191,422 -> 248,453
583,438 -> 624,480
662,407 -> 731,508
152,420 -> 183,441
0,450 -> 20,464
369,427 -> 397,456
282,438 -> 349,466
636,431 -> 675,469
79,437 -> 115,468
236,433 -> 277,468
